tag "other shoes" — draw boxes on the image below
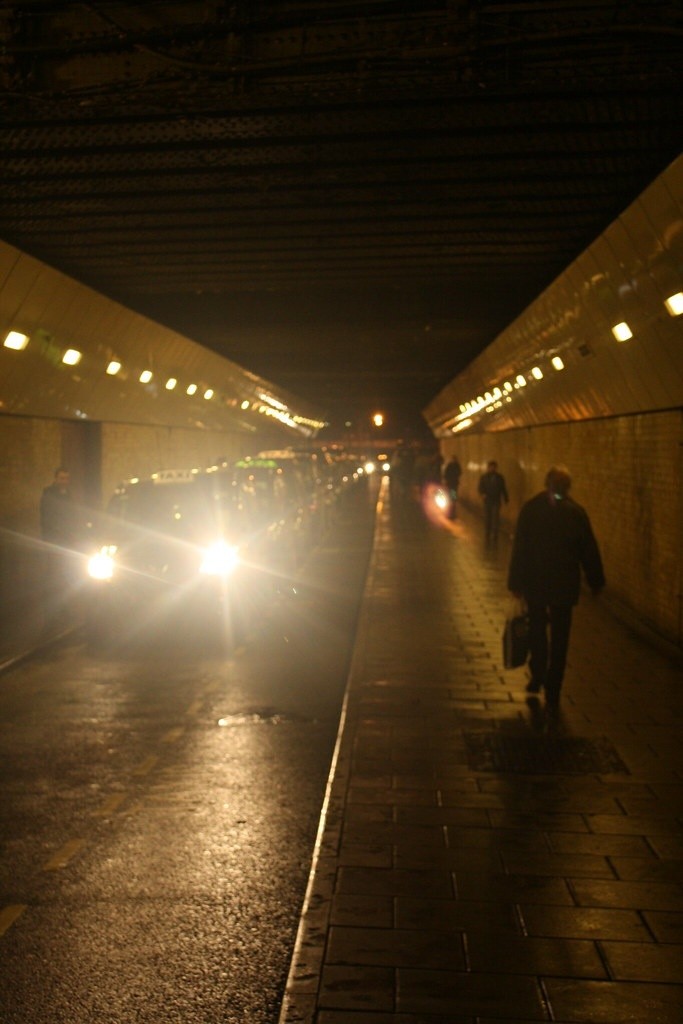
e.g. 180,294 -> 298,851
525,673 -> 547,694
546,691 -> 560,718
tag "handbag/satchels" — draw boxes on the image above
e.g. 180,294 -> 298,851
503,593 -> 528,668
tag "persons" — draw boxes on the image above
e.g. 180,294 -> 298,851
443,455 -> 462,519
41,467 -> 76,572
506,465 -> 606,714
479,460 -> 510,545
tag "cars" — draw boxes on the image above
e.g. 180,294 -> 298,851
98,437 -> 369,632
